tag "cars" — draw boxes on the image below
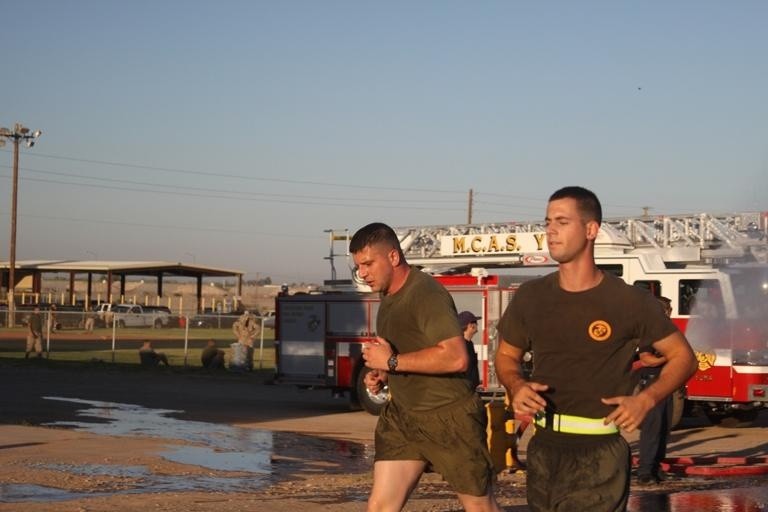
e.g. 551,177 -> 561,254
93,302 -> 113,329
261,309 -> 275,328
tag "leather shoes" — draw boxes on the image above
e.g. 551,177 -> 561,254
637,473 -> 659,485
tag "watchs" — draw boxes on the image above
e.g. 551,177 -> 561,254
387,353 -> 397,375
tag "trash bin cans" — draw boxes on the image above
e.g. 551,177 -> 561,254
229,343 -> 253,371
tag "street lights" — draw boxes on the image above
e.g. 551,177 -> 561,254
0,121 -> 41,329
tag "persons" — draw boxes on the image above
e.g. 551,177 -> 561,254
138,340 -> 169,370
638,295 -> 675,486
232,314 -> 261,345
458,310 -> 482,385
24,307 -> 44,359
200,338 -> 226,369
85,305 -> 95,335
49,304 -> 57,333
493,185 -> 698,511
350,222 -> 502,511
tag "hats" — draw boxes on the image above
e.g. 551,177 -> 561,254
457,311 -> 482,322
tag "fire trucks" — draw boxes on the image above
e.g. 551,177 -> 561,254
275,213 -> 768,431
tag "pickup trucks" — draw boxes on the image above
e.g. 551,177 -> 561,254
108,301 -> 169,329
